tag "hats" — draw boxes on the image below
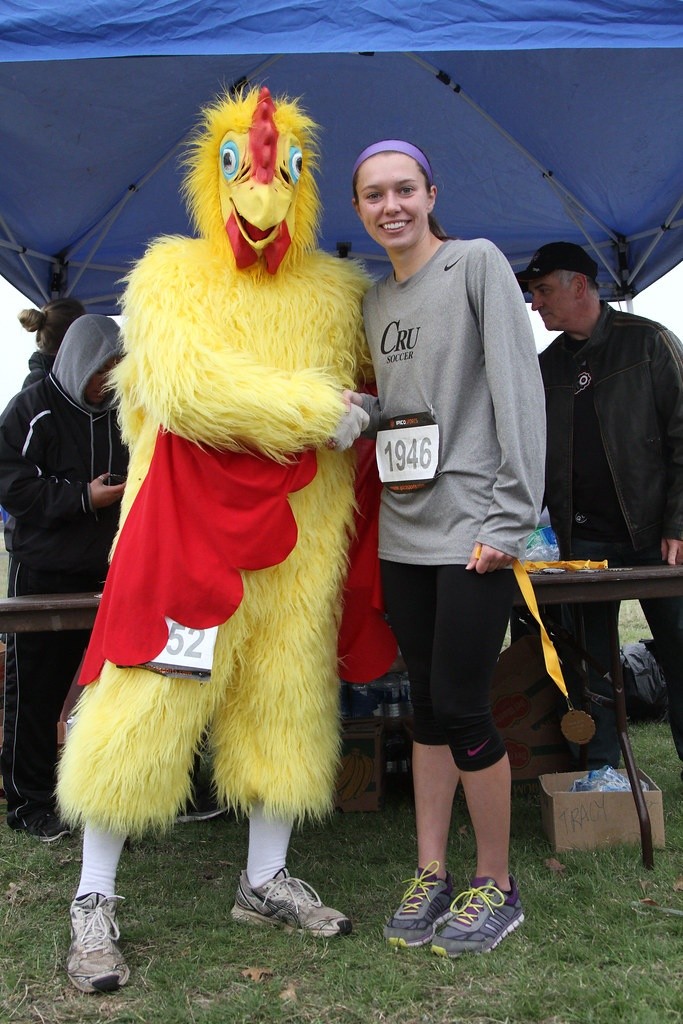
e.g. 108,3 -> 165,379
514,242 -> 599,292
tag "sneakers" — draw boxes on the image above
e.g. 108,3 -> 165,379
383,867 -> 459,948
432,877 -> 525,957
66,891 -> 129,993
25,812 -> 71,843
175,795 -> 233,823
230,867 -> 351,938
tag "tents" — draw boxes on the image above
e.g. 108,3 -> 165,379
1,0 -> 683,315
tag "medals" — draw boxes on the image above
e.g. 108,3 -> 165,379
561,698 -> 596,745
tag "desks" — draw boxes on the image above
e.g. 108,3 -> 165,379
1,563 -> 683,871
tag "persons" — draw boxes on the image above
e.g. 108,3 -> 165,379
323,138 -> 548,959
511,242 -> 682,775
1,316 -> 230,843
1,297 -> 85,572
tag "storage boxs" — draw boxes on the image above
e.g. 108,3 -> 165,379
332,716 -> 388,813
487,634 -> 572,781
536,767 -> 666,854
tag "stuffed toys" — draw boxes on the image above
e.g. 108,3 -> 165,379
53,85 -> 377,996
